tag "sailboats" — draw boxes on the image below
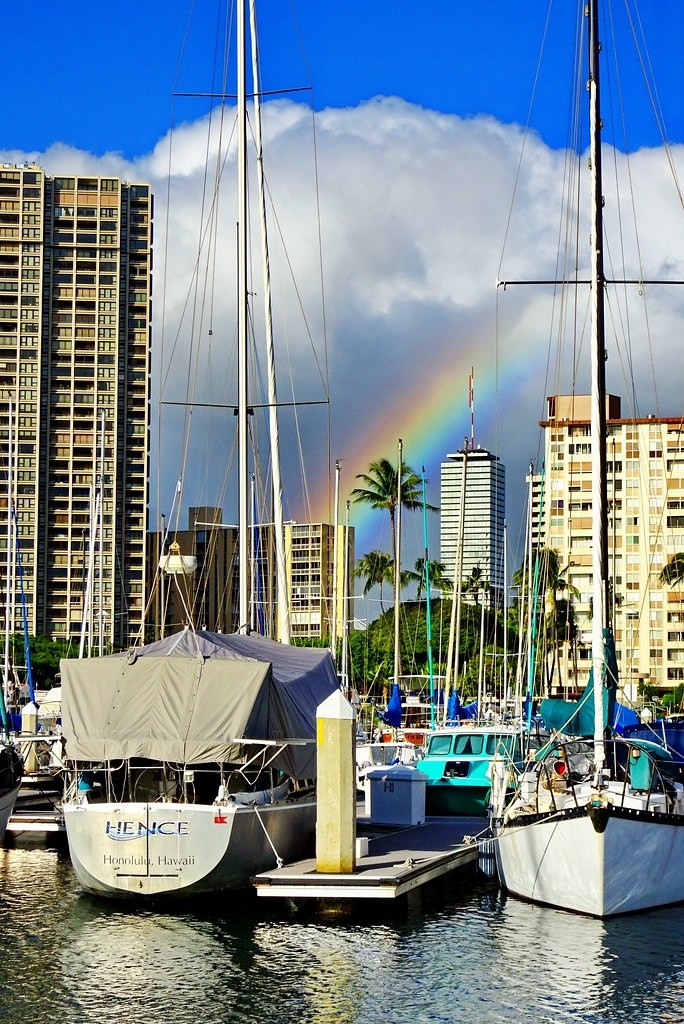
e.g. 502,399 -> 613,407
2,0 -> 684,924
